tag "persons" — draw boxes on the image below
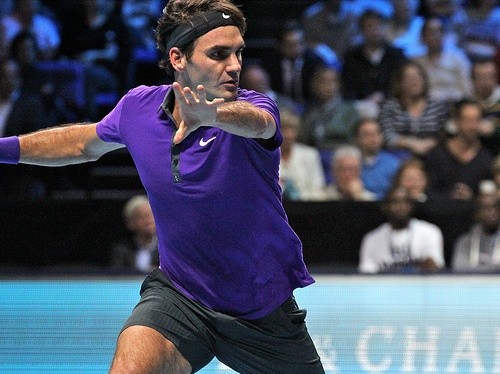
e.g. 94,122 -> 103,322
418,0 -> 499,62
346,10 -> 411,103
450,190 -> 500,274
322,146 -> 376,201
381,63 -> 457,153
379,1 -> 427,54
304,70 -> 359,142
358,188 -> 444,272
0,0 -> 330,374
308,0 -> 355,62
392,162 -> 441,207
461,62 -> 500,134
425,101 -> 498,195
407,17 -> 470,98
327,118 -> 398,201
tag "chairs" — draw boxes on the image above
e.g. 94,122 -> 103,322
0,0 -> 166,195
